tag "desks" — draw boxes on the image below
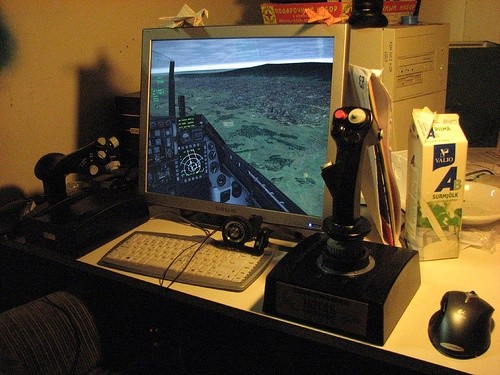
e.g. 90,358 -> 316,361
0,146 -> 500,375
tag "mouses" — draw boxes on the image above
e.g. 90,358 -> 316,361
428,291 -> 495,360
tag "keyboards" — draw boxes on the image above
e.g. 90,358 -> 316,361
97,230 -> 273,289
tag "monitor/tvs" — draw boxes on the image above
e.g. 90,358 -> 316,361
137,21 -> 349,232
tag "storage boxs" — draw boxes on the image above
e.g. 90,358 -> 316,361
342,18 -> 451,152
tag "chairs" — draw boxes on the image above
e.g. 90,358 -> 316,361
0,292 -> 101,375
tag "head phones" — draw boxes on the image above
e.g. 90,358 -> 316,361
221,215 -> 273,255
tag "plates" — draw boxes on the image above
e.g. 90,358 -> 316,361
462,180 -> 500,227
